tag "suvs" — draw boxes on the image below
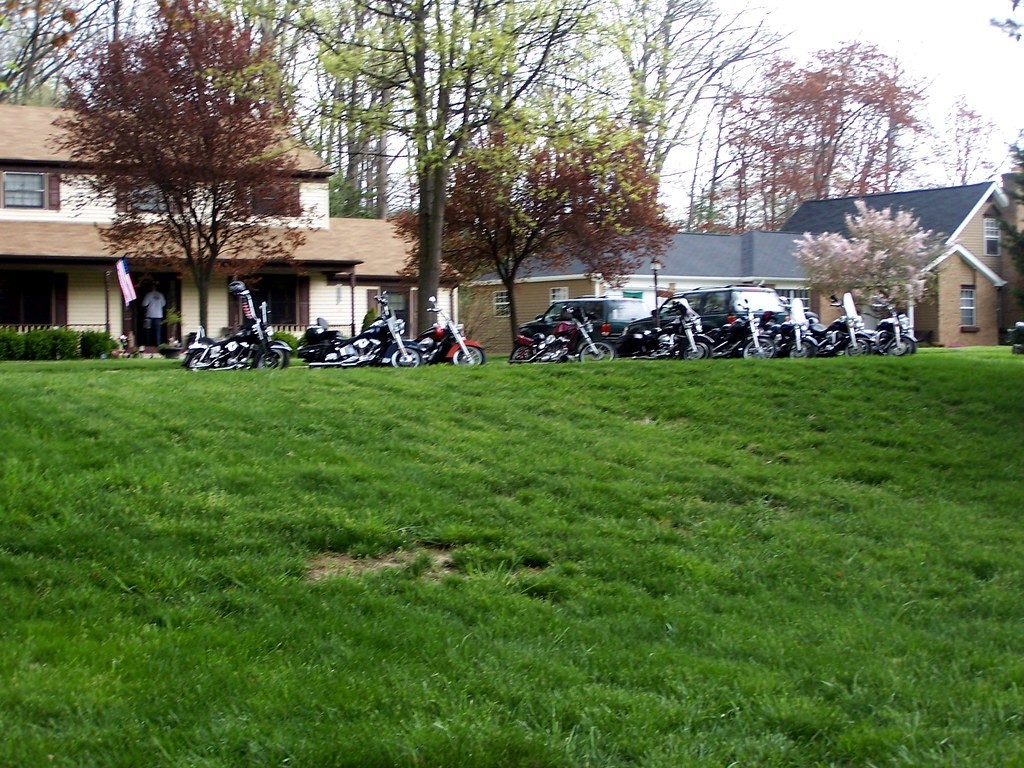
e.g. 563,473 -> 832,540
652,282 -> 791,341
536,294 -> 654,354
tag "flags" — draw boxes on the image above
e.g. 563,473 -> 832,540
116,257 -> 137,307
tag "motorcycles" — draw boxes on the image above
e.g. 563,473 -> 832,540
296,290 -> 426,370
181,291 -> 293,373
507,302 -> 618,365
612,299 -> 716,362
835,302 -> 919,357
674,298 -> 776,361
401,296 -> 486,367
784,293 -> 878,360
731,297 -> 819,360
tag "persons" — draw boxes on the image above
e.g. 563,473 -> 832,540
142,286 -> 166,346
